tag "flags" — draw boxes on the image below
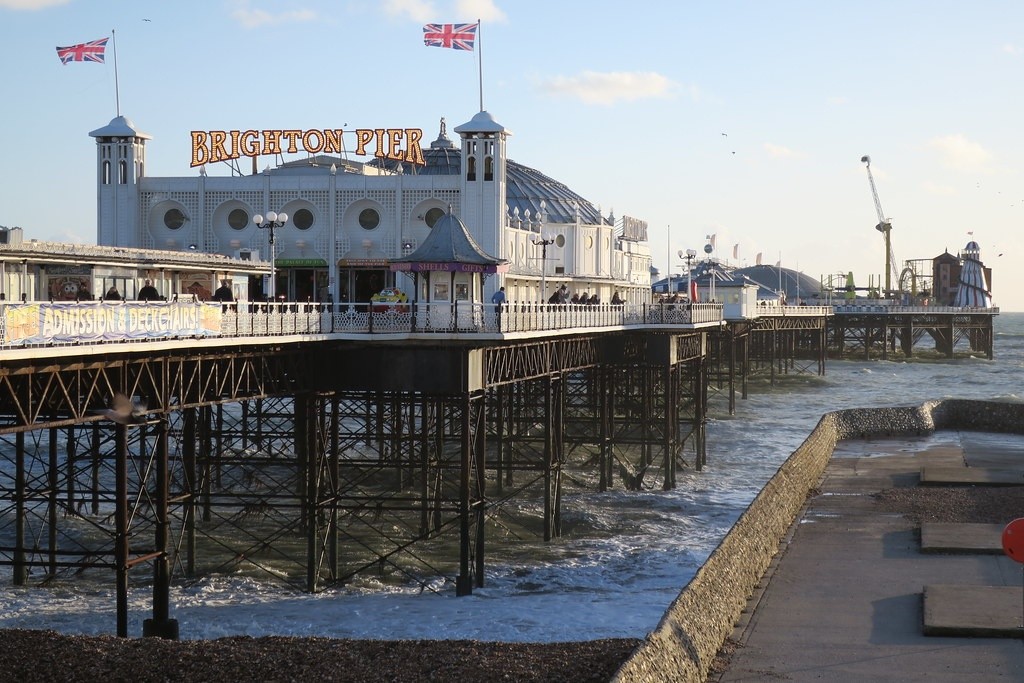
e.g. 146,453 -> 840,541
422,22 -> 478,51
56,38 -> 109,64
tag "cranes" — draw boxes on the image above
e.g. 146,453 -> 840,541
862,155 -> 906,292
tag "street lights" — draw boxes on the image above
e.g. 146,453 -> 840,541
703,258 -> 719,301
678,249 -> 697,304
703,269 -> 716,301
529,233 -> 559,304
251,211 -> 289,302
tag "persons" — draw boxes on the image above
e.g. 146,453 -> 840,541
610,291 -> 625,306
74,283 -> 92,301
548,285 -> 570,304
105,287 -> 121,300
570,293 -> 579,304
138,278 -> 161,301
578,292 -> 589,304
491,287 -> 506,324
758,300 -> 807,307
589,294 -> 598,304
214,281 -> 234,302
669,293 -> 679,305
